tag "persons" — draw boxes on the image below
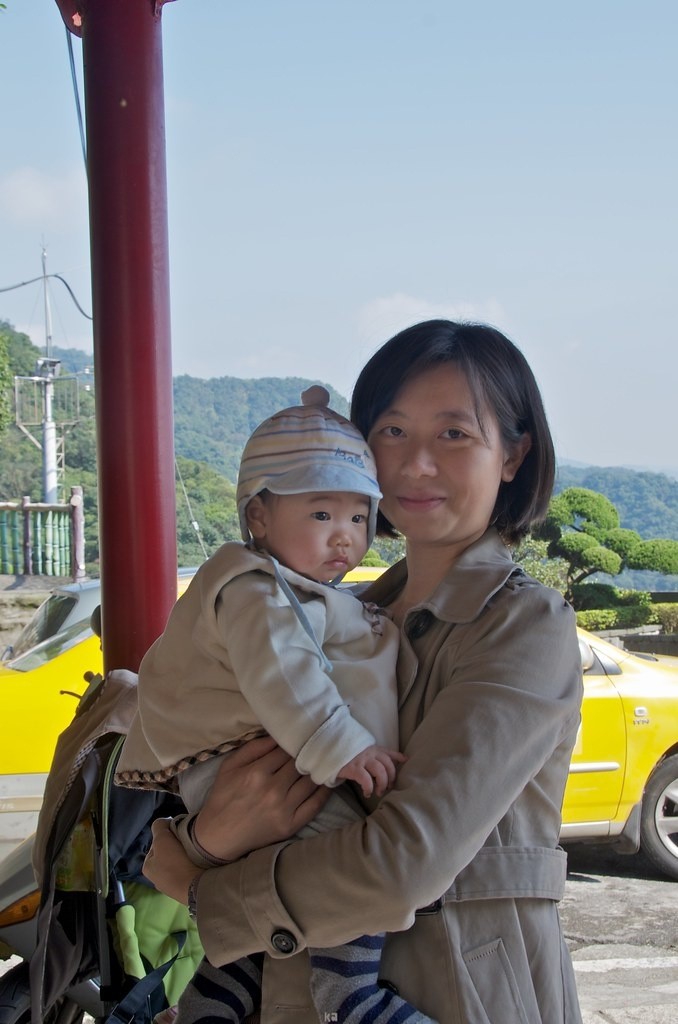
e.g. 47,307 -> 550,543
114,387 -> 437,1024
142,321 -> 584,1024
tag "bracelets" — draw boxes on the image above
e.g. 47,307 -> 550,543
188,874 -> 198,921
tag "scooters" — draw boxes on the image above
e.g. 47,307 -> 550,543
0,671 -> 110,1023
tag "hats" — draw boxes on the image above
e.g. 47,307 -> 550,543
236,385 -> 383,672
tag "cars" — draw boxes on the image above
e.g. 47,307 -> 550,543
0,566 -> 677,884
